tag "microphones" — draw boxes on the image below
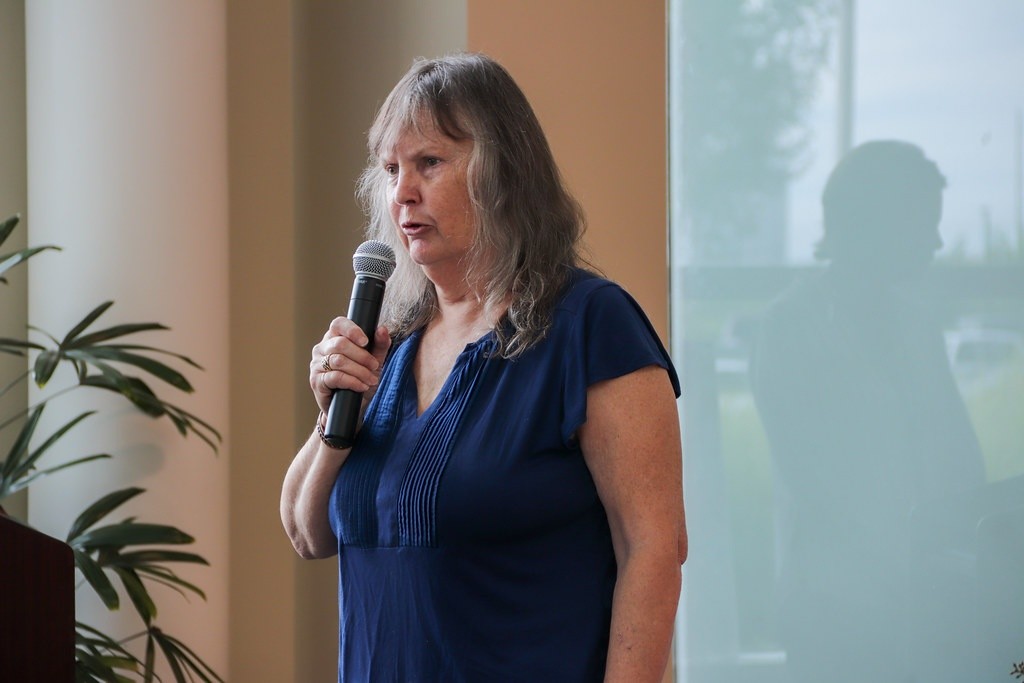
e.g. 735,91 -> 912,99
324,239 -> 397,449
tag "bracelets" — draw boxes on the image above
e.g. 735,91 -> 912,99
317,412 -> 353,449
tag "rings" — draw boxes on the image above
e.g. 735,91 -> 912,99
322,354 -> 332,371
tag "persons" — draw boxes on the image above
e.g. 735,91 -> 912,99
280,52 -> 688,683
